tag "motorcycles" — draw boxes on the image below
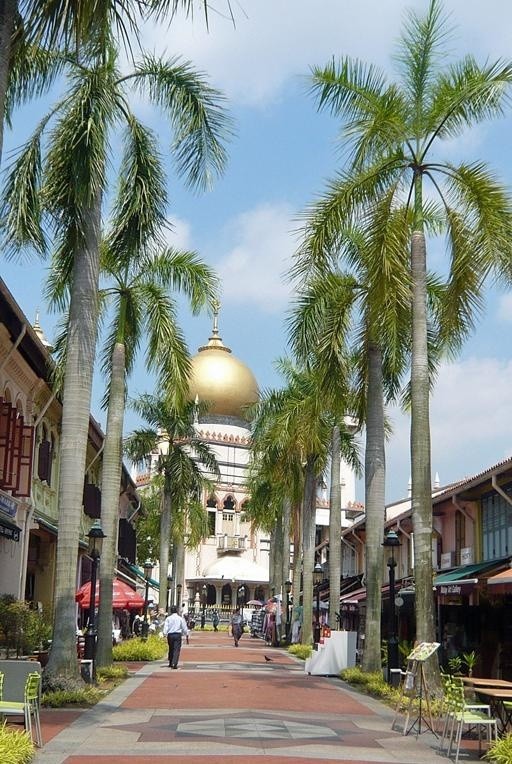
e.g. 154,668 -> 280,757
183,614 -> 198,629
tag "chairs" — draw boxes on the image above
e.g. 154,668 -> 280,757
1,671 -> 49,750
77,632 -> 97,659
438,672 -> 503,764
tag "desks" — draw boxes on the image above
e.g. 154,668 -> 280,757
453,668 -> 511,741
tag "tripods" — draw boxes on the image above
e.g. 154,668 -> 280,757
406,661 -> 439,740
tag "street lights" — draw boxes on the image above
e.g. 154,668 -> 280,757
165,574 -> 173,612
284,578 -> 293,636
312,560 -> 324,651
141,562 -> 153,641
380,526 -> 403,683
83,518 -> 107,664
239,585 -> 245,623
200,583 -> 208,628
177,584 -> 182,613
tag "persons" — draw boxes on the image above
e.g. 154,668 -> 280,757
75,603 -> 220,669
226,608 -> 462,671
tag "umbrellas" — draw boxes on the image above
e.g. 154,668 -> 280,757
74,577 -> 145,610
245,599 -> 264,605
